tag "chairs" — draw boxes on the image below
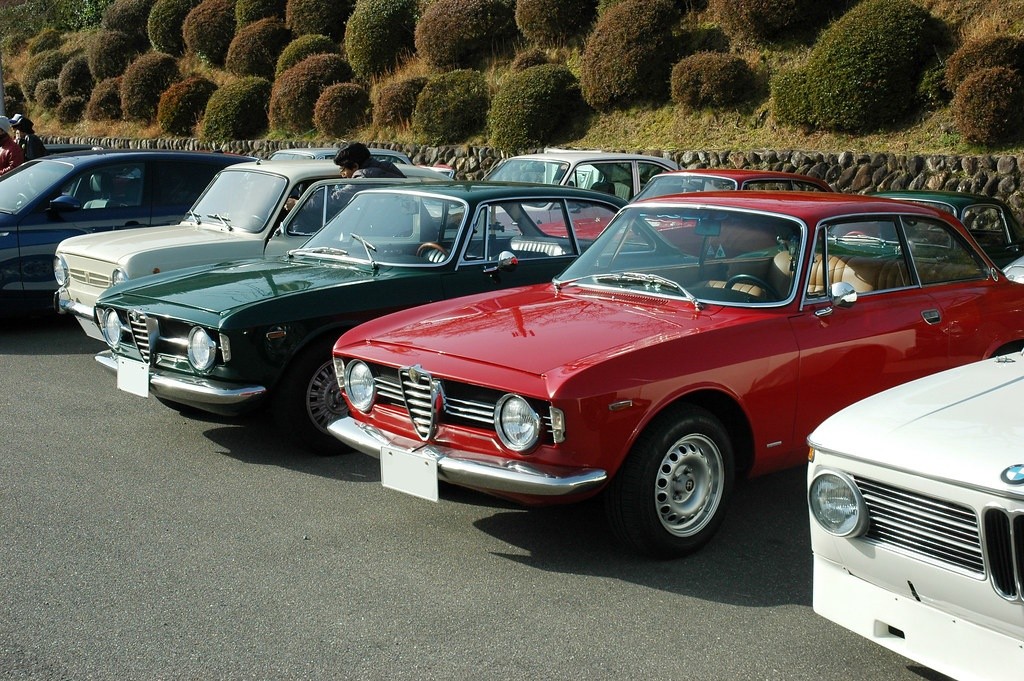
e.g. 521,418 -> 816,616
694,280 -> 763,298
423,249 -> 447,262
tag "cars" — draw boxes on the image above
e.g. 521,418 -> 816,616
421,152 -> 720,232
525,169 -> 843,262
0,147 -> 273,315
51,161 -> 518,345
93,182 -> 705,459
265,148 -> 455,216
805,361 -> 1024,681
733,189 -> 1021,298
319,189 -> 1022,556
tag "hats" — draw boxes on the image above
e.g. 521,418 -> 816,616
9,113 -> 22,124
0,116 -> 14,139
12,117 -> 34,134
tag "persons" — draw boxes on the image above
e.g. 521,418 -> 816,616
0,114 -> 50,175
284,142 -> 438,242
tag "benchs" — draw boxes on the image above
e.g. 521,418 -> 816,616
769,250 -> 974,299
510,235 -> 589,257
